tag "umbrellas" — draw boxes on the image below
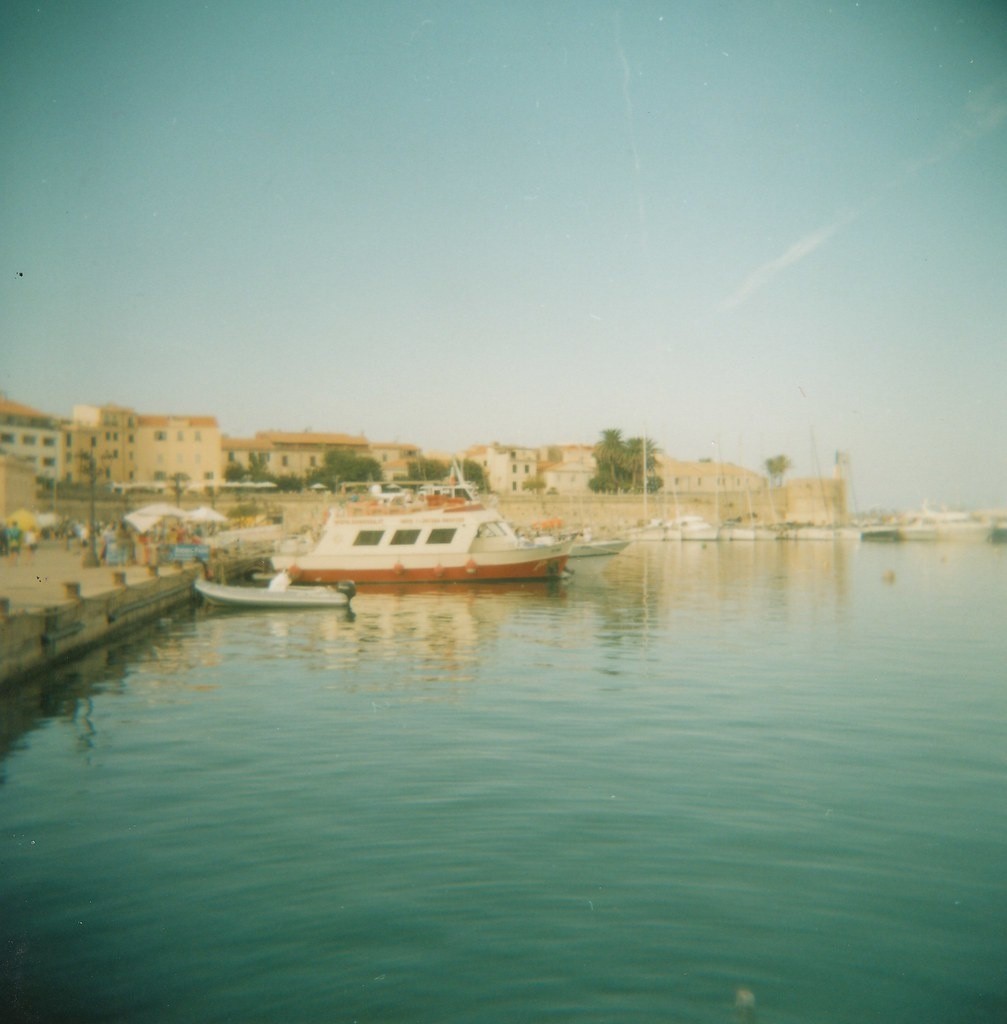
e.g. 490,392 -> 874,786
262,480 -> 278,490
310,482 -> 324,496
128,502 -> 184,543
186,507 -> 227,533
386,484 -> 400,495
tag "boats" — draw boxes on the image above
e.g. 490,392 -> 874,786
265,492 -> 582,584
193,564 -> 356,609
518,517 -> 631,556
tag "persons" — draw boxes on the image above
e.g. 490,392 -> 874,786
3,514 -> 272,571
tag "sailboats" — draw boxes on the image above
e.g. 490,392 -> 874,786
615,411 -> 1006,546
